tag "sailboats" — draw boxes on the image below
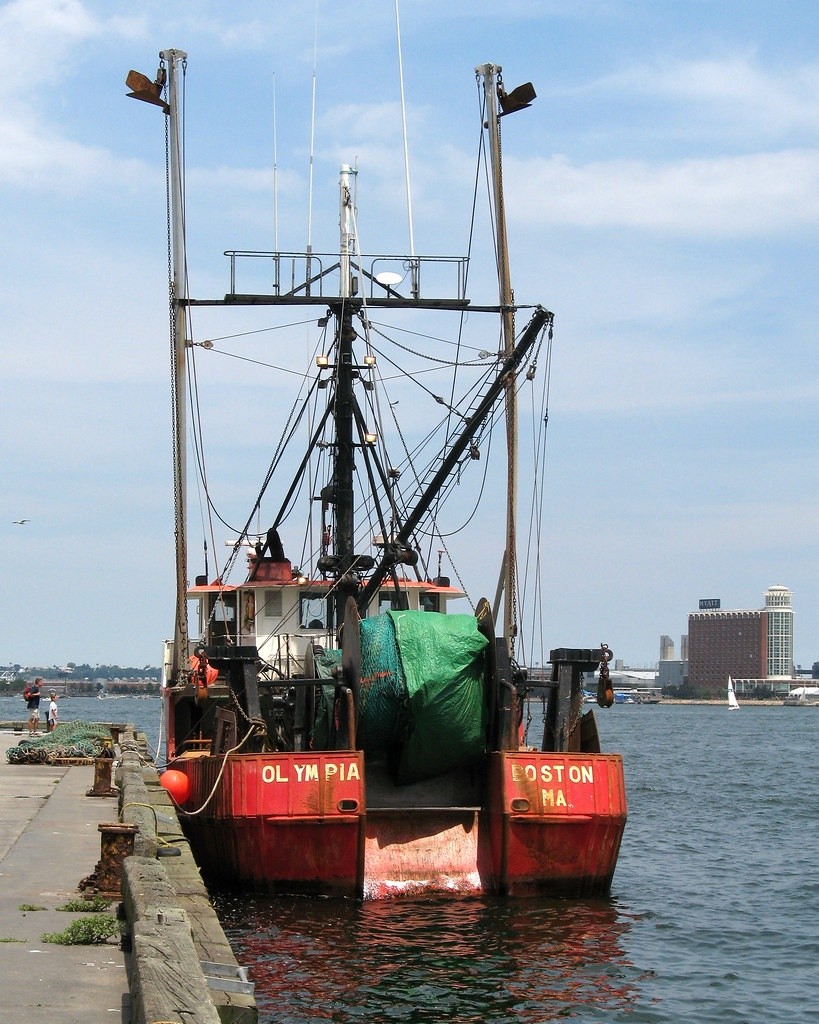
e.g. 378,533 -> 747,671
728,675 -> 740,710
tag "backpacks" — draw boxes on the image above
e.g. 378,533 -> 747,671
23,687 -> 33,701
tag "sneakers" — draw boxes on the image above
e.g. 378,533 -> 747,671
29,732 -> 40,737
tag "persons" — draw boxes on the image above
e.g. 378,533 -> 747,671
27,677 -> 43,737
48,693 -> 58,733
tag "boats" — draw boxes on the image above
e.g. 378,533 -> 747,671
121,0 -> 628,903
624,698 -> 634,704
584,696 -> 595,703
41,697 -> 51,702
